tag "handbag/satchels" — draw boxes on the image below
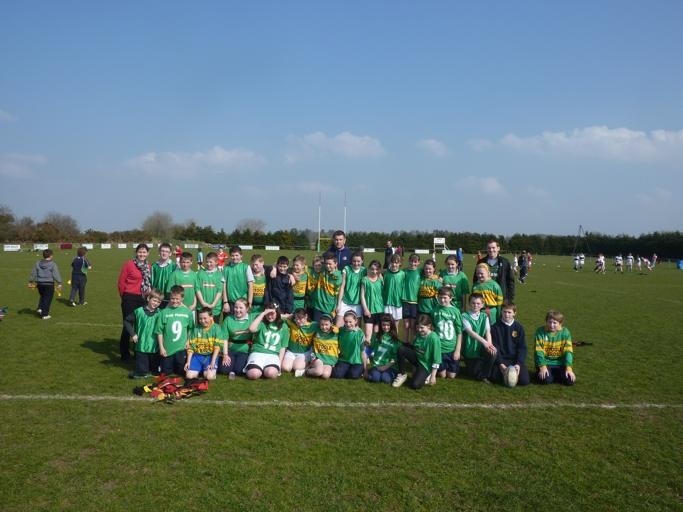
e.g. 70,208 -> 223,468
81,265 -> 88,276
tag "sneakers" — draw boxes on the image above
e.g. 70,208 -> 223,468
517,278 -> 526,284
391,373 -> 407,388
36,309 -> 51,320
122,356 -> 132,364
69,301 -> 88,307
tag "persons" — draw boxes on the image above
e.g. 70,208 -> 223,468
28,248 -> 61,320
69,246 -> 92,306
531,307 -> 578,385
571,251 -> 657,274
